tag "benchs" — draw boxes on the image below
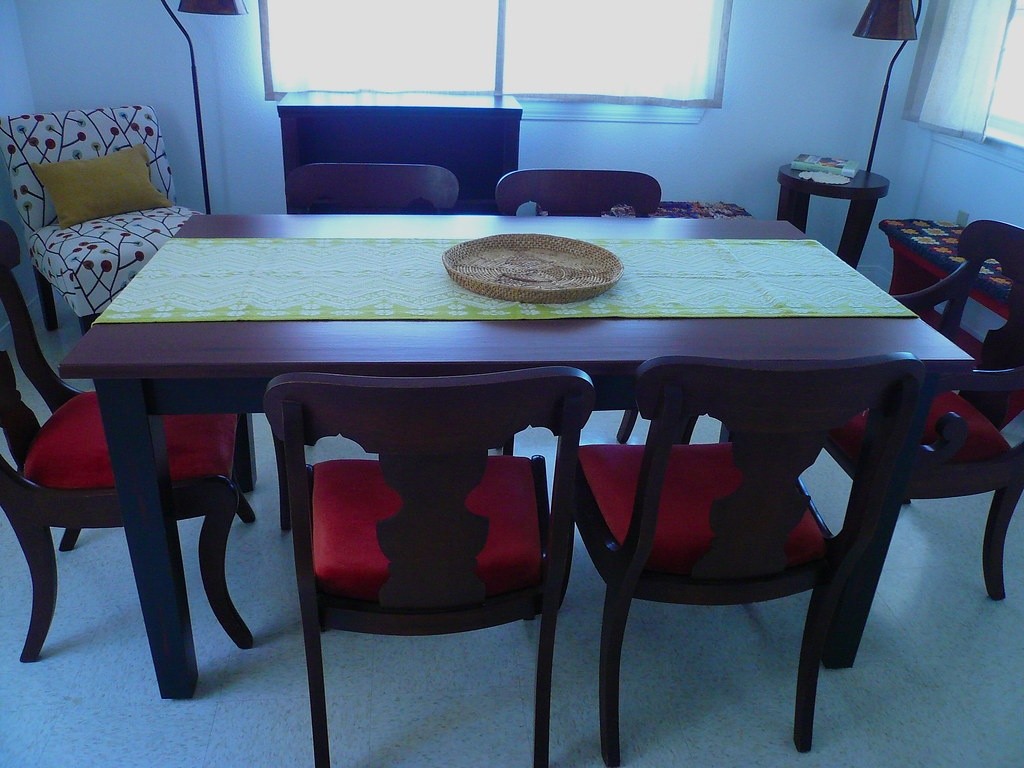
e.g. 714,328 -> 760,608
649,202 -> 755,220
879,219 -> 1024,430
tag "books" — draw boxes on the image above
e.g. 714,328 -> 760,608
791,154 -> 859,178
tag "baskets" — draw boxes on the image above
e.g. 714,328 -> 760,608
441,233 -> 624,303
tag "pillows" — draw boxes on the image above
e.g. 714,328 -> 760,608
32,144 -> 173,231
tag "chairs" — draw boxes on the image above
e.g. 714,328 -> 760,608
260,360 -> 596,768
818,220 -> 1024,599
270,160 -> 459,532
0,221 -> 255,662
494,168 -> 663,443
561,356 -> 923,768
0,106 -> 211,337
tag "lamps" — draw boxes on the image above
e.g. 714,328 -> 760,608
852,0 -> 925,172
161,0 -> 250,212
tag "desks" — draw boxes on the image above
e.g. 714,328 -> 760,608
58,214 -> 977,701
276,91 -> 522,216
776,163 -> 889,268
